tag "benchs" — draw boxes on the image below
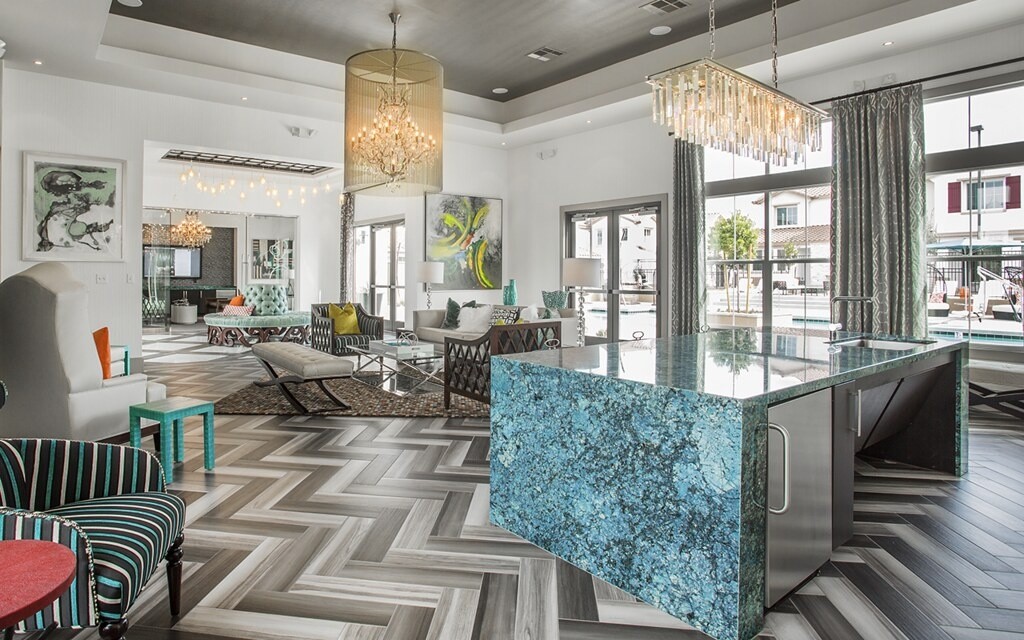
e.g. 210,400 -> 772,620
203,310 -> 313,347
968,358 -> 1024,421
412,304 -> 552,351
250,341 -> 354,414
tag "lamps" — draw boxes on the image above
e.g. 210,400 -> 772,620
343,11 -> 443,198
647,1 -> 828,168
414,260 -> 444,308
286,268 -> 295,296
169,209 -> 213,251
563,257 -> 602,347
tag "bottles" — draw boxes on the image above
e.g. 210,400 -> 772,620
506,279 -> 517,306
503,286 -> 509,305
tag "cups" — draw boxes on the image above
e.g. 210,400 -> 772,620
955,332 -> 963,339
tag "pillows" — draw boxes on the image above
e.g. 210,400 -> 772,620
440,298 -> 561,333
222,297 -> 257,316
328,301 -> 361,336
93,327 -> 113,379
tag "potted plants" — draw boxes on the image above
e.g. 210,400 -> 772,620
707,210 -> 763,326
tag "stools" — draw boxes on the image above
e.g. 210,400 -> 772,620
129,395 -> 216,485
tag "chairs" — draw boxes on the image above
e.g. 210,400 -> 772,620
444,320 -> 563,411
0,262 -> 186,639
310,302 -> 384,366
204,289 -> 235,315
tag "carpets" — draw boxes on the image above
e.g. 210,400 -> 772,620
197,352 -> 491,417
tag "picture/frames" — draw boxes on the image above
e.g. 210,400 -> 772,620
246,215 -> 299,283
21,149 -> 127,263
424,191 -> 503,292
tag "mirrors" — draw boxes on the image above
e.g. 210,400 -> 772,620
142,243 -> 203,280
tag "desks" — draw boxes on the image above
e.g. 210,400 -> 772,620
170,304 -> 198,324
0,539 -> 76,640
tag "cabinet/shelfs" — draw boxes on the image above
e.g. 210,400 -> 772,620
764,349 -> 958,610
143,289 -> 232,315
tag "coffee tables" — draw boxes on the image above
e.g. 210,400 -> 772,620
345,344 -> 453,397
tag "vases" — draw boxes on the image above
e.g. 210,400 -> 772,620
503,278 -> 516,305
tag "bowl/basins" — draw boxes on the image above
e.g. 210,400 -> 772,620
541,290 -> 569,310
